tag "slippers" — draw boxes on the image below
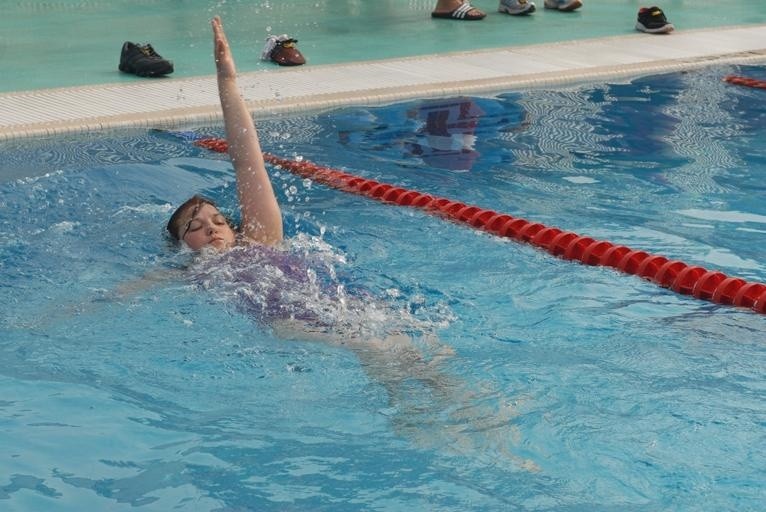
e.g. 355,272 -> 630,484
430,3 -> 487,20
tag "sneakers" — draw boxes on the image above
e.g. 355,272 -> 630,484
118,41 -> 174,76
543,0 -> 582,12
268,36 -> 305,65
634,6 -> 674,33
498,0 -> 536,15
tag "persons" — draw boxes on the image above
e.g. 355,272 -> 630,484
6,13 -> 545,482
431,0 -> 486,17
